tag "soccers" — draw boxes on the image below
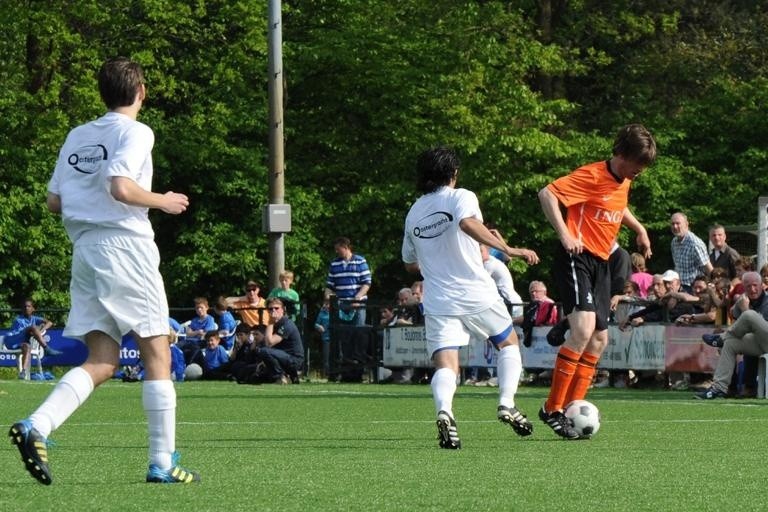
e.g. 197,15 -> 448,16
185,363 -> 203,378
563,401 -> 601,439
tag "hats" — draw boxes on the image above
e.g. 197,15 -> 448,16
660,269 -> 680,282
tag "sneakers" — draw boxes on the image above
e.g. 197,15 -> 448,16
8,420 -> 54,486
145,463 -> 200,483
464,368 -> 691,388
701,332 -> 724,348
436,410 -> 460,450
497,403 -> 534,436
539,402 -> 579,440
692,389 -> 726,400
30,371 -> 56,381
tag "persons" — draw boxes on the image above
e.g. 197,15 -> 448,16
400,147 -> 542,450
8,56 -> 191,486
3,299 -> 64,381
538,124 -> 653,438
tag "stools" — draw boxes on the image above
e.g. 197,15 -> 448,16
755,353 -> 768,399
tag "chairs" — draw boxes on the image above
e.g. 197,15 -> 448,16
0,327 -> 47,383
